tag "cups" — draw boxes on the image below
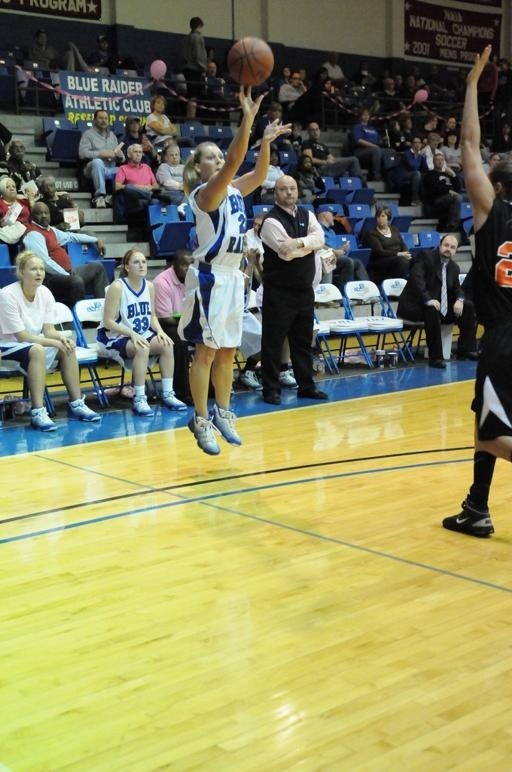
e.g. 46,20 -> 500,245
387,352 -> 398,368
28,192 -> 36,208
375,350 -> 385,369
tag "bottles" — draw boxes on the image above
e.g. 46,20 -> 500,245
316,358 -> 325,379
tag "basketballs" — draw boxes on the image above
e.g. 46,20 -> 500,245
228,37 -> 275,86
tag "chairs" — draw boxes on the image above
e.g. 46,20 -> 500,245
0,41 -> 510,424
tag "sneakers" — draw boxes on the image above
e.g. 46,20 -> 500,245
456,348 -> 482,361
176,202 -> 188,217
278,370 -> 299,390
188,411 -> 221,455
428,357 -> 447,368
238,368 -> 264,390
29,406 -> 59,432
297,388 -> 328,400
65,392 -> 103,422
131,395 -> 156,418
442,502 -> 496,537
209,403 -> 243,447
263,393 -> 282,405
159,391 -> 188,410
95,194 -> 113,208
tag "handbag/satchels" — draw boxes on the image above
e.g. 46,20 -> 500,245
0,220 -> 27,245
382,148 -> 401,171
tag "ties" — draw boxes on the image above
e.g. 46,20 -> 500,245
439,262 -> 448,317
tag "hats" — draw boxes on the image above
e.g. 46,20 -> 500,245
125,115 -> 141,122
315,204 -> 338,217
97,34 -> 108,43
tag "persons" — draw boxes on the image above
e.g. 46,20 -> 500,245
29,28 -> 123,70
183,17 -> 235,126
366,206 -> 479,368
442,44 -> 511,538
279,53 -> 511,233
1,93 -> 191,431
237,101 -> 368,403
182,85 -> 292,456
